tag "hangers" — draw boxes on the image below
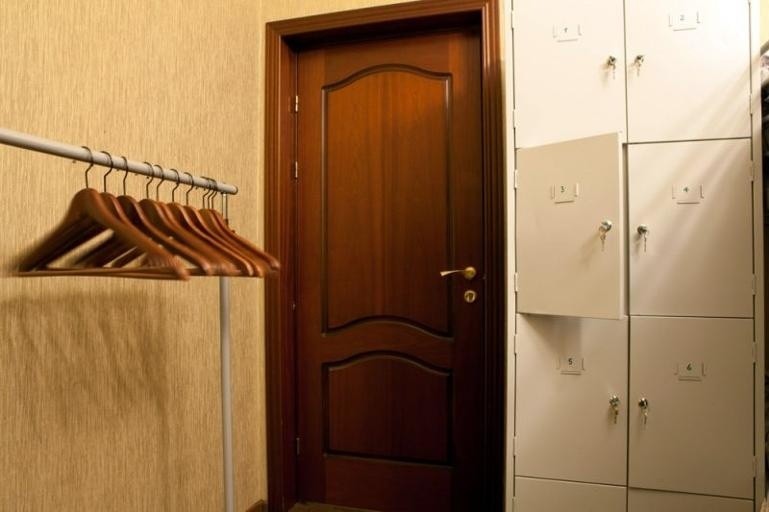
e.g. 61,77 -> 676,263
16,131 -> 278,280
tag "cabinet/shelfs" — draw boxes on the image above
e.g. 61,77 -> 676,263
500,0 -> 764,509
